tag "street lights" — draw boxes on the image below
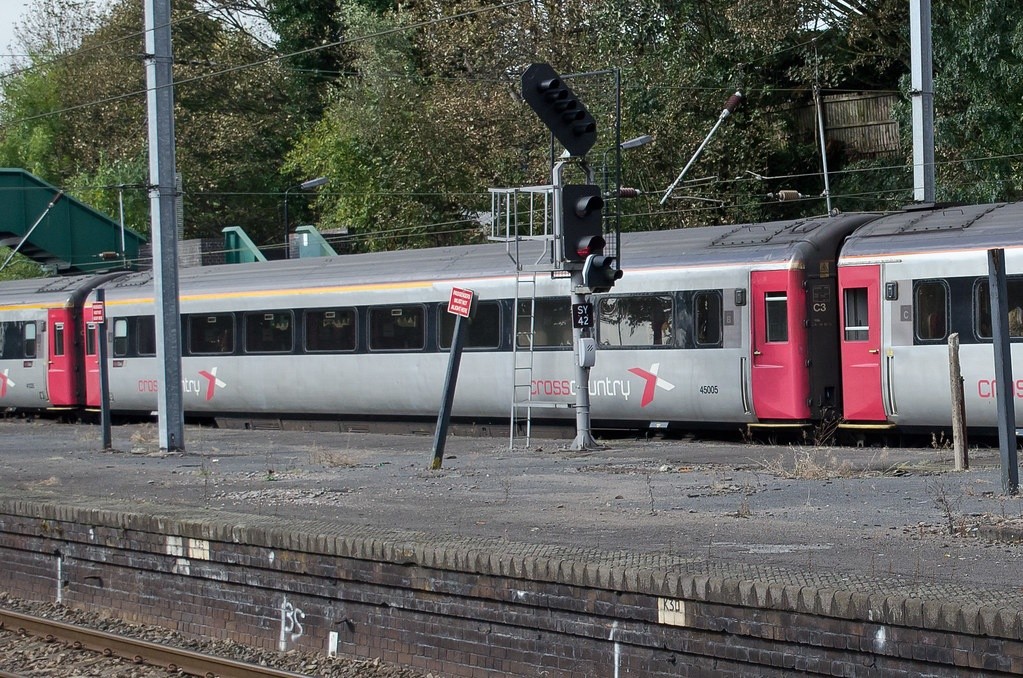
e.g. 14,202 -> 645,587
603,135 -> 656,234
283,178 -> 331,260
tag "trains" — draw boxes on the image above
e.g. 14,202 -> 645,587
0,200 -> 1023,445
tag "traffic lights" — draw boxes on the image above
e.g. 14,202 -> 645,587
521,59 -> 598,157
562,183 -> 616,296
